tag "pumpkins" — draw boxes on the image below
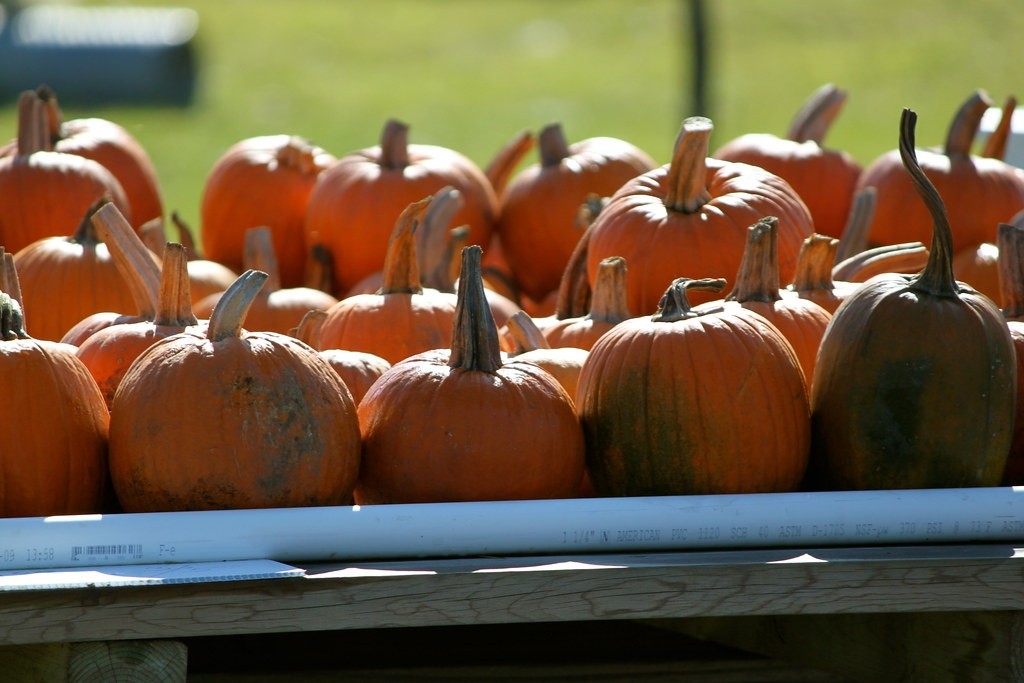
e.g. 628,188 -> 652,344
0,84 -> 1024,521
